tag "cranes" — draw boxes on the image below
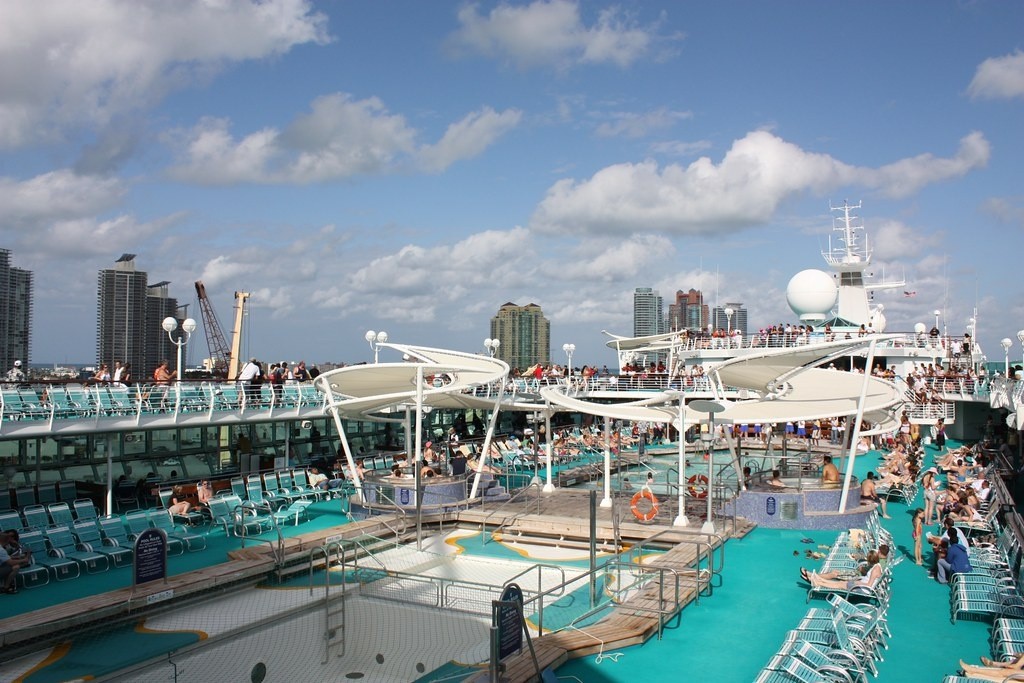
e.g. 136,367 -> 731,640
195,281 -> 242,379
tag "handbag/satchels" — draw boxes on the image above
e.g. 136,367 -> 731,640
235,374 -> 239,382
269,373 -> 274,380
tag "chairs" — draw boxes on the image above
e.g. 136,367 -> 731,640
440,423 -> 640,471
754,507 -> 905,683
0,454 -> 394,593
940,443 -> 1024,664
875,465 -> 926,506
941,674 -> 1024,683
0,380 -> 346,422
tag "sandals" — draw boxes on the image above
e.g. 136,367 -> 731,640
0,586 -> 16,594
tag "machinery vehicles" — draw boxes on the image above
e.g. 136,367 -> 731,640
227,291 -> 250,384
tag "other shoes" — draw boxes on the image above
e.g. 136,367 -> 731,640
793,551 -> 799,556
803,550 -> 826,560
183,522 -> 197,527
926,566 -> 938,578
333,494 -> 341,498
800,538 -> 815,543
818,544 -> 831,549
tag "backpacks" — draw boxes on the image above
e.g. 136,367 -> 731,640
154,367 -> 164,384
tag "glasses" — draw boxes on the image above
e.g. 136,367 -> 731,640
104,366 -> 108,369
202,483 -> 208,485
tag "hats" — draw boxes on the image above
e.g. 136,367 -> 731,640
312,363 -> 316,367
926,467 -> 939,476
510,436 -> 517,439
249,357 -> 256,362
426,442 -> 432,447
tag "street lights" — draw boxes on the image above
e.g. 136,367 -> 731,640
365,331 -> 388,363
563,344 -> 575,384
934,310 -> 940,330
877,304 -> 884,333
725,309 -> 733,349
162,316 -> 196,405
1000,339 -> 1012,378
484,338 -> 500,396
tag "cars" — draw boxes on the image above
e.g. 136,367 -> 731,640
195,453 -> 217,465
152,446 -> 168,451
95,439 -> 108,450
191,437 -> 201,443
162,458 -> 180,466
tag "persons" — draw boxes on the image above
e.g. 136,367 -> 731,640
4,360 -> 25,390
822,456 -> 840,483
391,419 -> 678,485
236,433 -> 251,455
679,323 -> 874,350
502,362 -> 705,392
93,361 -> 178,416
309,459 -> 375,502
0,529 -> 32,595
309,426 -> 322,453
800,545 -> 889,596
623,472 -> 653,490
743,467 -> 785,489
709,416 -> 870,447
826,362 -> 896,384
960,653 -> 1024,683
113,470 -> 214,516
908,325 -> 1024,408
685,451 -> 710,466
861,410 -> 991,596
237,357 -> 320,408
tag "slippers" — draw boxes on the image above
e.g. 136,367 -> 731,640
800,567 -> 811,584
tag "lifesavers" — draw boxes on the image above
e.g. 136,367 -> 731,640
630,491 -> 659,520
687,474 -> 708,498
135,387 -> 149,400
